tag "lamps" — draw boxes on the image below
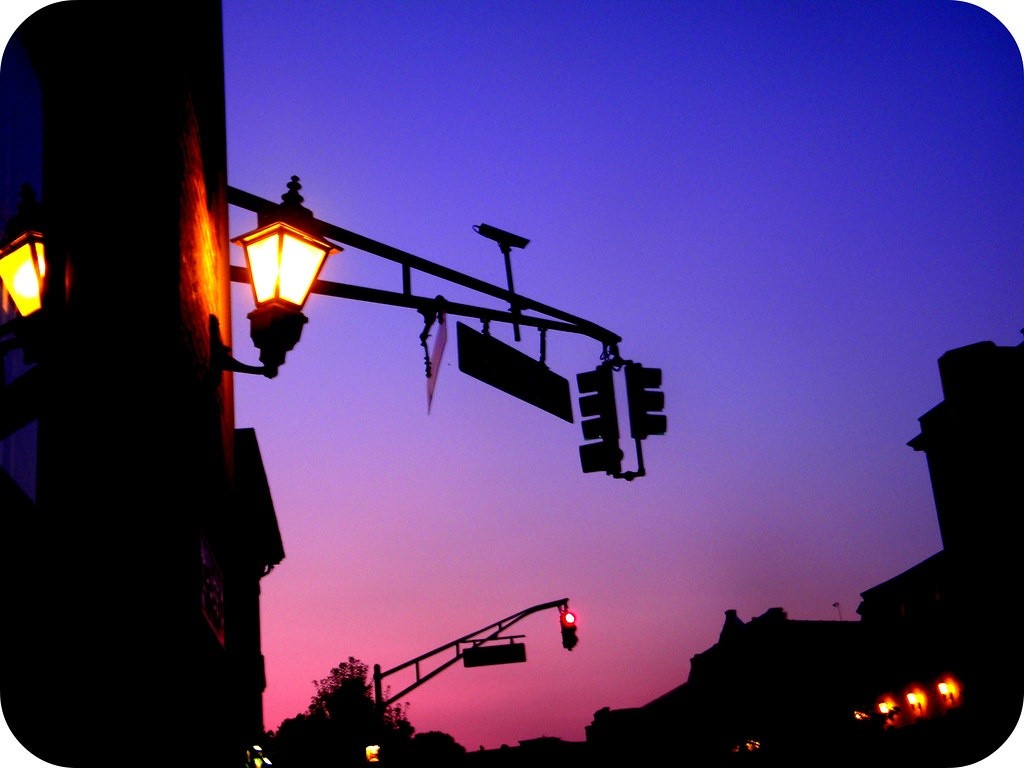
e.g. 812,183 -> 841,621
1,179 -> 53,340
208,175 -> 345,389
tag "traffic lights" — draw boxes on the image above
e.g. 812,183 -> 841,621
623,360 -> 669,440
559,611 -> 579,652
571,363 -> 624,477
